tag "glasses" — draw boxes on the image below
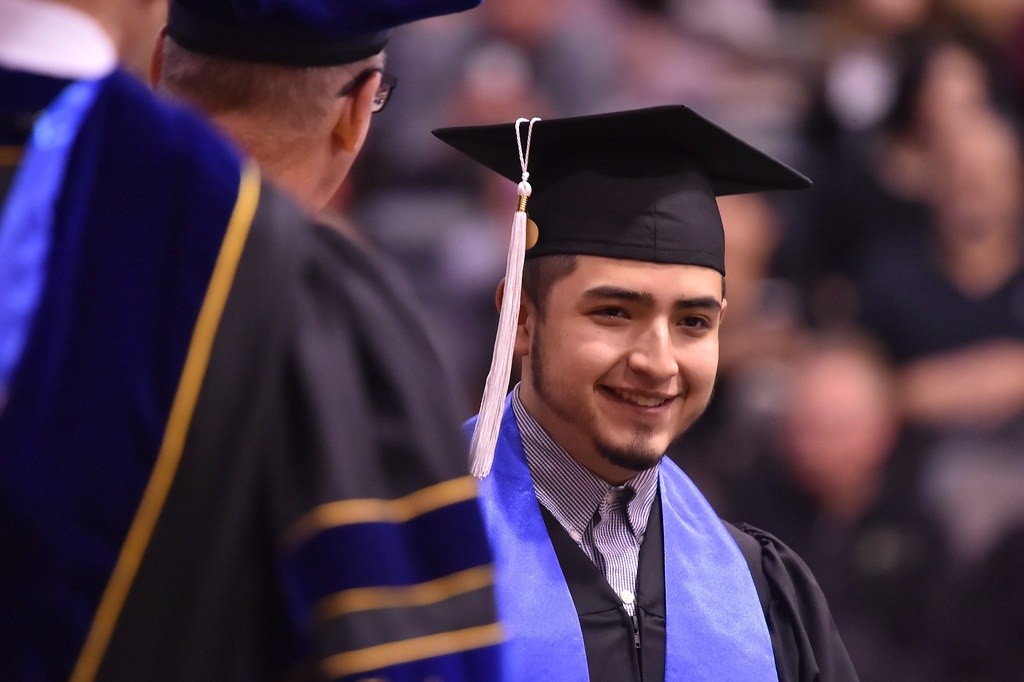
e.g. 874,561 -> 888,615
334,66 -> 399,113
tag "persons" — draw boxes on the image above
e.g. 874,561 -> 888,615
420,0 -> 1024,631
0,66 -> 514,682
148,0 -> 483,217
429,104 -> 860,682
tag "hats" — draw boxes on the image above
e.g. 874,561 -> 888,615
161,0 -> 479,69
429,100 -> 817,481
